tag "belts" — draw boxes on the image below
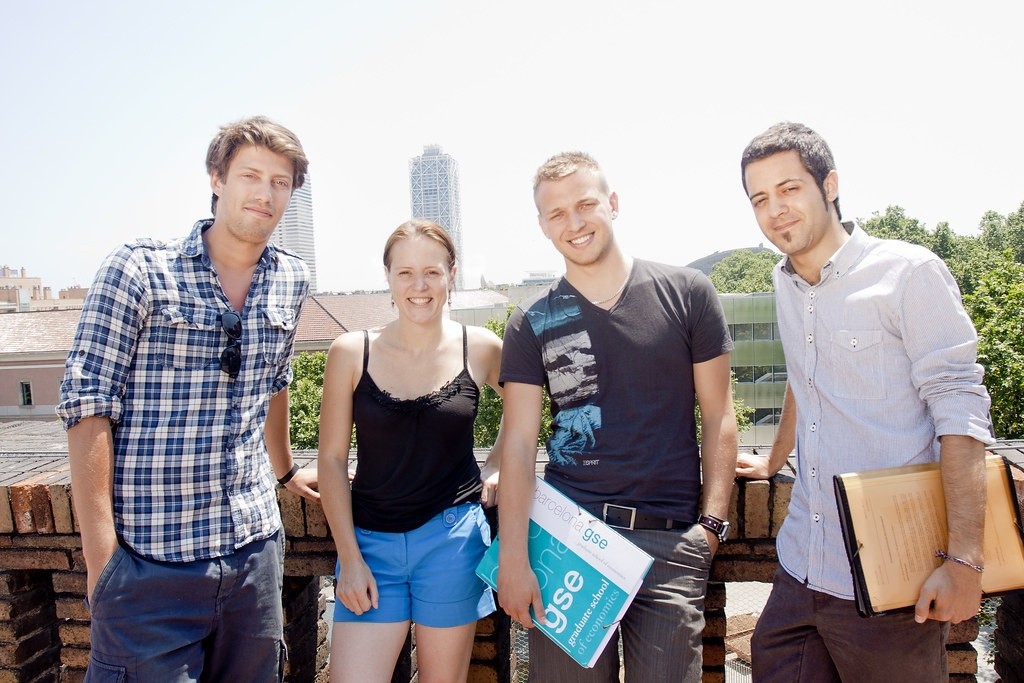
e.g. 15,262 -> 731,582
573,501 -> 696,532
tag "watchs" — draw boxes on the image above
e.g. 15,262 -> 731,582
698,513 -> 732,543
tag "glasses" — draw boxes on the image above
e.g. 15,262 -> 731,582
219,312 -> 243,378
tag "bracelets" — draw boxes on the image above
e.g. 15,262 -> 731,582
934,549 -> 984,574
277,462 -> 299,486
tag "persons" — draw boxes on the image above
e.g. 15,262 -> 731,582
496,150 -> 739,683
314,218 -> 516,683
735,119 -> 998,683
56,115 -> 356,683
